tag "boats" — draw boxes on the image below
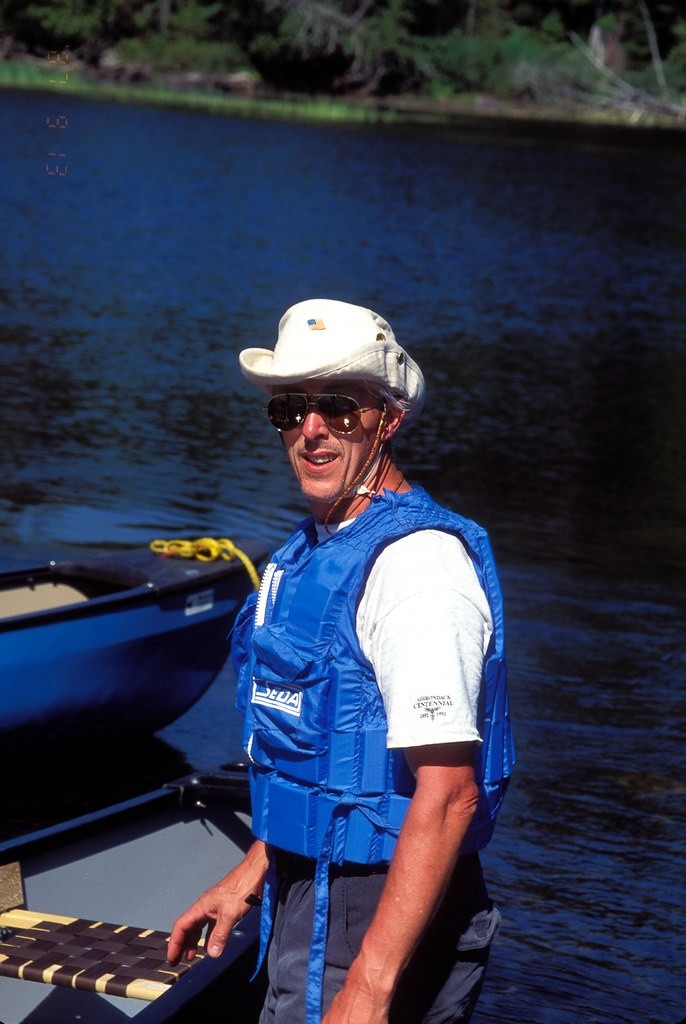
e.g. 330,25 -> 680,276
1,758 -> 281,1023
0,534 -> 281,784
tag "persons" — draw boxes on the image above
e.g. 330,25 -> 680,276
166,299 -> 516,1024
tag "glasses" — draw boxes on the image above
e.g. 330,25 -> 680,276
262,394 -> 382,433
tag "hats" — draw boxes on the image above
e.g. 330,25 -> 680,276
238,299 -> 426,401
